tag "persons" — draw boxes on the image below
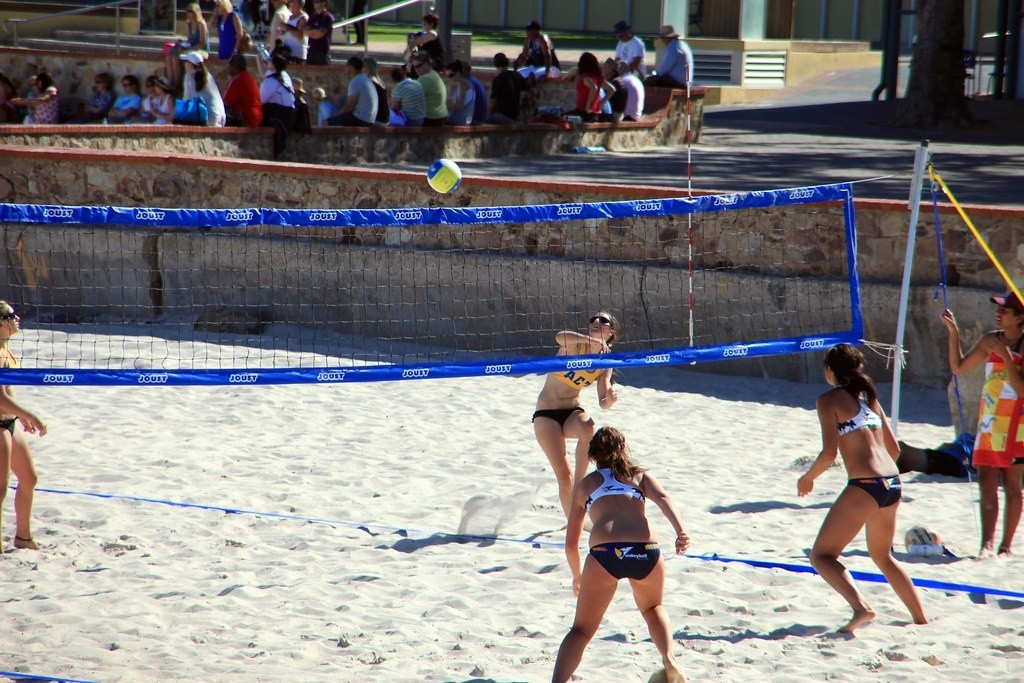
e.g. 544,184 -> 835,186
0,300 -> 48,554
939,290 -> 1024,559
798,344 -> 928,633
533,311 -> 618,530
0,0 -> 694,130
551,426 -> 689,683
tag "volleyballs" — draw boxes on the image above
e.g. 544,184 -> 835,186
426,158 -> 463,194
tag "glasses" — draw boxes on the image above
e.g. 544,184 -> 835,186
122,83 -> 133,87
414,63 -> 425,68
447,72 -> 455,79
589,317 -> 612,328
146,85 -> 153,88
96,81 -> 104,84
1,311 -> 16,320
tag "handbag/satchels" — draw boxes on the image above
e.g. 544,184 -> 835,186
163,41 -> 176,57
172,96 -> 208,125
295,100 -> 312,136
529,105 -> 582,125
388,107 -> 407,126
225,108 -> 242,127
233,11 -> 253,53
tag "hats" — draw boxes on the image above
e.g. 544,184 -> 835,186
524,21 -> 541,31
991,291 -> 1024,310
154,75 -> 175,90
655,25 -> 680,37
290,76 -> 306,94
605,58 -> 620,72
311,87 -> 326,99
612,20 -> 633,34
179,53 -> 204,66
